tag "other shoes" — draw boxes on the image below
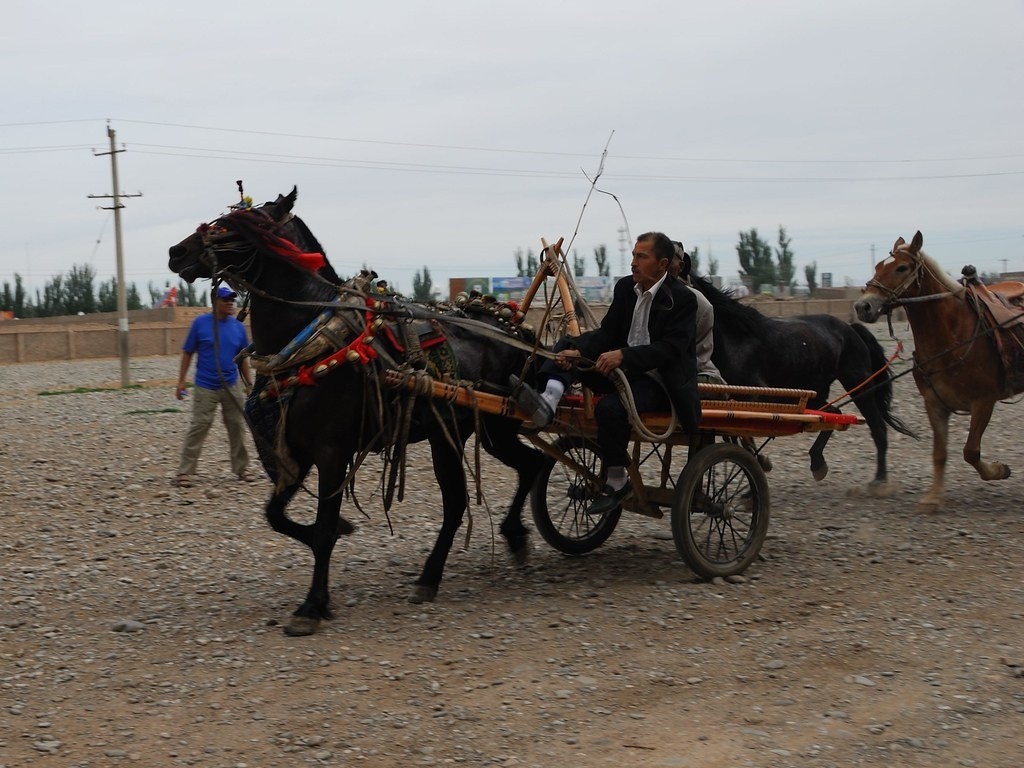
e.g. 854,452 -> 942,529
175,475 -> 193,487
239,470 -> 256,481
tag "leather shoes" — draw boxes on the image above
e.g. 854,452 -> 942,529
587,478 -> 634,515
509,374 -> 555,428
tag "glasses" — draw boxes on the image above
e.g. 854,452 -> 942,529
217,297 -> 235,303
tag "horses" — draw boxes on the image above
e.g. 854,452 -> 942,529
678,230 -> 1024,514
168,184 -> 538,635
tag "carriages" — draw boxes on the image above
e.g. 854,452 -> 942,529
167,178 -> 867,638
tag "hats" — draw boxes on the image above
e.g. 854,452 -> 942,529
671,241 -> 684,261
211,287 -> 237,303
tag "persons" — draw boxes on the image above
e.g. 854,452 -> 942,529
510,233 -> 729,515
173,287 -> 256,488
